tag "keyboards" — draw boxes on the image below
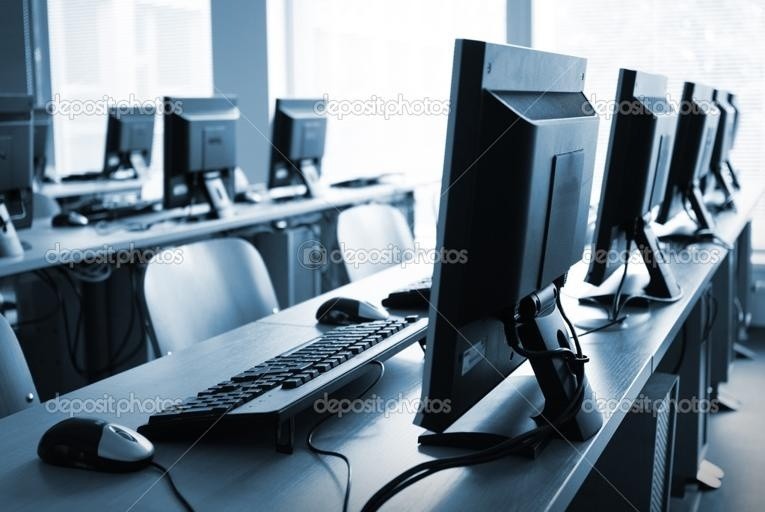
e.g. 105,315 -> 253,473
137,315 -> 429,455
381,270 -> 434,310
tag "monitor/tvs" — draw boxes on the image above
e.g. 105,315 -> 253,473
411,38 -> 602,457
165,96 -> 241,224
0,96 -> 157,258
587,68 -> 741,311
265,97 -> 329,203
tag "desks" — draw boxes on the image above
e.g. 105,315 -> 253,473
0,171 -> 755,511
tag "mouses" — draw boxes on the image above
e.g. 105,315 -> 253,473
36,417 -> 154,472
234,190 -> 261,203
316,297 -> 389,324
